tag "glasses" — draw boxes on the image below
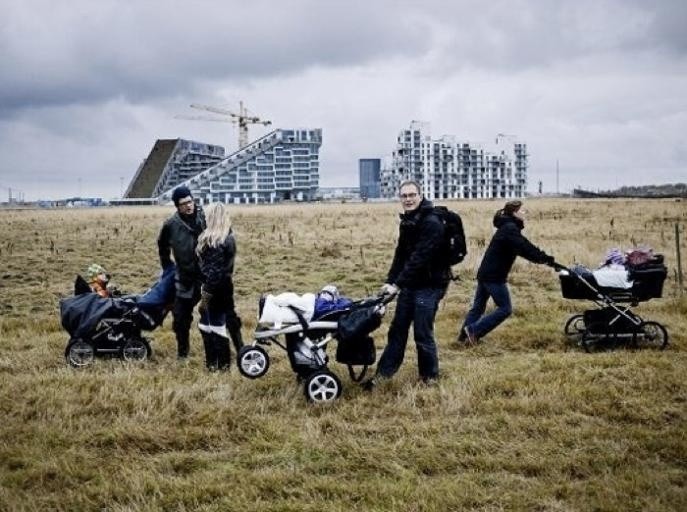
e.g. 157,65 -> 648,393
400,192 -> 420,199
178,199 -> 193,206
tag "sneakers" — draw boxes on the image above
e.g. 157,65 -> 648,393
463,322 -> 480,349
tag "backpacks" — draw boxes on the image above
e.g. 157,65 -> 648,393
430,205 -> 467,267
336,336 -> 376,365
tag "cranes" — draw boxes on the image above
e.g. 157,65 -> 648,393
175,101 -> 272,150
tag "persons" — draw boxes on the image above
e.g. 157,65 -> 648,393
369,179 -> 452,380
158,184 -> 245,358
311,284 -> 354,321
190,200 -> 231,373
458,199 -> 555,346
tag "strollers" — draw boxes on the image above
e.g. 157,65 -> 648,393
236,288 -> 397,406
547,255 -> 668,352
58,263 -> 176,370
